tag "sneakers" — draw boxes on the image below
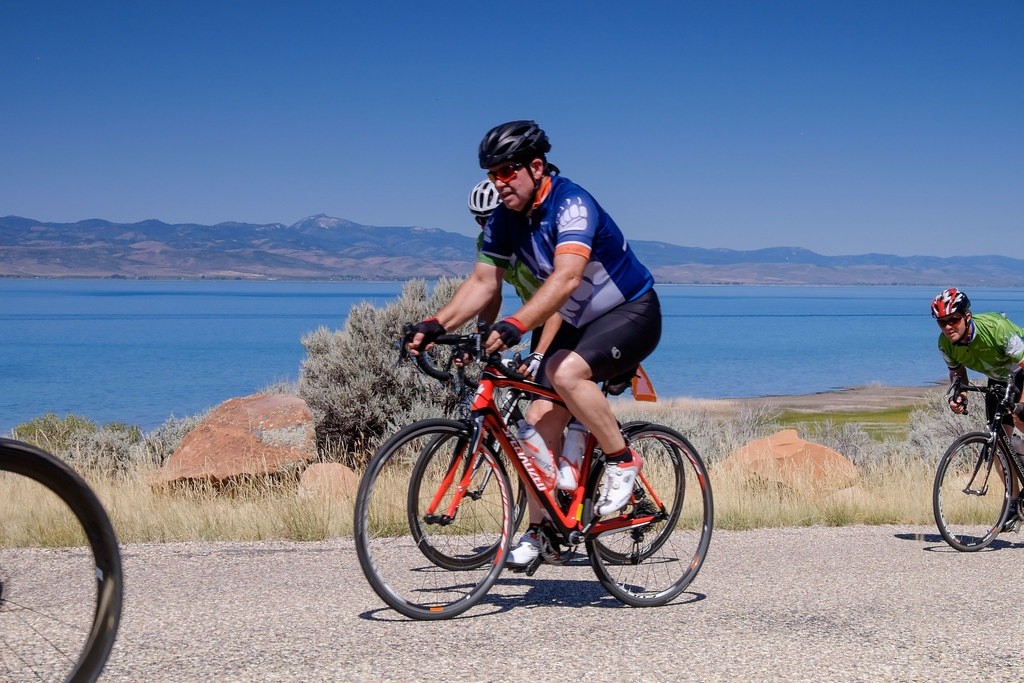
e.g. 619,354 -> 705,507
594,447 -> 643,516
505,530 -> 548,565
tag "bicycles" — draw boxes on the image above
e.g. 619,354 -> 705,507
931,367 -> 1024,552
0,437 -> 125,683
350,321 -> 715,621
406,333 -> 687,570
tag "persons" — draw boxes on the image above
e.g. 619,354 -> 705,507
402,118 -> 664,573
930,289 -> 1024,532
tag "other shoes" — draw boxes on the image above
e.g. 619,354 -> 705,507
1002,499 -> 1019,530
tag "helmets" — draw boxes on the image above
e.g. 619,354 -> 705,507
931,288 -> 971,319
479,119 -> 551,169
467,178 -> 505,217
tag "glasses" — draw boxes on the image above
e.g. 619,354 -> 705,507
475,216 -> 489,224
937,314 -> 963,328
487,163 -> 522,182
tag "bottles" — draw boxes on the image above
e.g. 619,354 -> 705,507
518,419 -> 561,490
556,421 -> 587,490
1011,433 -> 1024,466
